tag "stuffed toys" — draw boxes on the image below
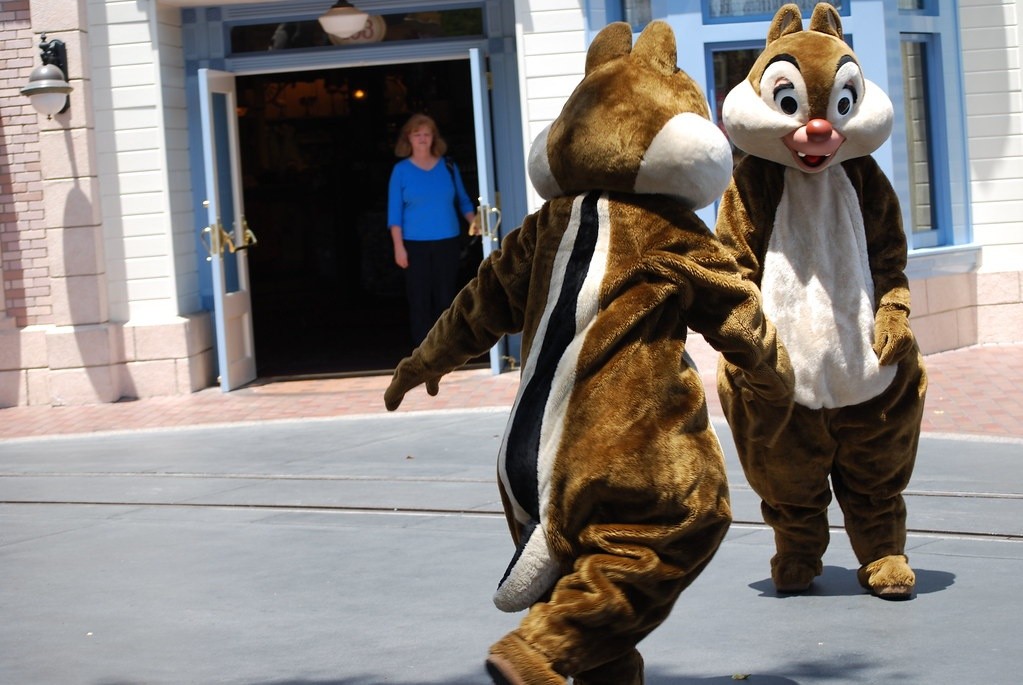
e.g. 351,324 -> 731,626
711,2 -> 929,597
385,19 -> 795,685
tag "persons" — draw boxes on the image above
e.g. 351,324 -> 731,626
387,115 -> 475,340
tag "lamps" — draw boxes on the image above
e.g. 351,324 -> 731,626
20,31 -> 73,121
318,0 -> 369,39
